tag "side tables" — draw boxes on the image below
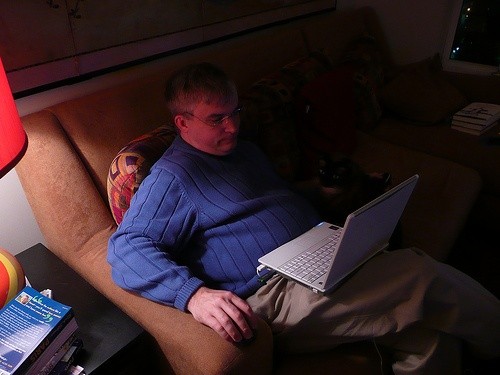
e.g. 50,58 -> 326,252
14,243 -> 176,375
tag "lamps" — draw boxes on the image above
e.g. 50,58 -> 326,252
0,57 -> 29,309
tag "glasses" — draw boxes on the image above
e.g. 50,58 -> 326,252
180,104 -> 241,128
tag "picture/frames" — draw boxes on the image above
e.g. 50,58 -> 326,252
0,0 -> 337,99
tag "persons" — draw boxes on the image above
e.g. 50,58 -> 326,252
106,62 -> 500,375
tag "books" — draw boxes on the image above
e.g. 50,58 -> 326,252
451,102 -> 500,136
0,285 -> 88,375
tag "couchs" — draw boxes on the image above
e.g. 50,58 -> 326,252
15,6 -> 500,375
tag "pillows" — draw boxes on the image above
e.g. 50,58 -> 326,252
329,35 -> 391,130
106,117 -> 178,226
384,54 -> 466,126
240,50 -> 340,183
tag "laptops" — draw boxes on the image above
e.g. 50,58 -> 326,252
258,173 -> 419,296
